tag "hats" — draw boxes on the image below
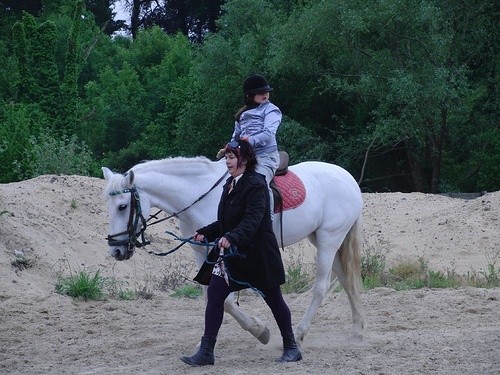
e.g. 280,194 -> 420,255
243,74 -> 274,95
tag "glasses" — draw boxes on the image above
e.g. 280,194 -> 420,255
227,141 -> 241,149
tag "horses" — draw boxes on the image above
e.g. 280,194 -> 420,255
101,155 -> 367,349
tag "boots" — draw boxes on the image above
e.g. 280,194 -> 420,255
181,336 -> 217,366
276,333 -> 303,362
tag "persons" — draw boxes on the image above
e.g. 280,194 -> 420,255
179,139 -> 304,367
216,75 -> 282,225
